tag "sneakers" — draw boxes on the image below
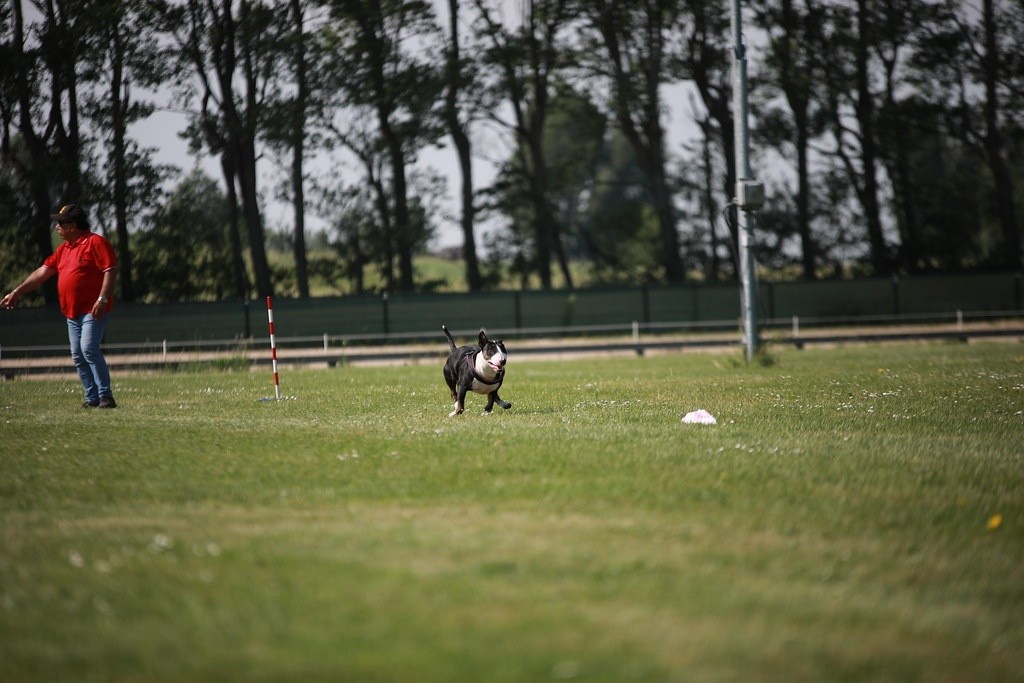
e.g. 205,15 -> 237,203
98,397 -> 118,408
80,402 -> 99,408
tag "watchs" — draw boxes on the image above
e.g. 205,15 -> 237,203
98,296 -> 107,302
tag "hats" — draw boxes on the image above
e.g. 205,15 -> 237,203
49,204 -> 88,222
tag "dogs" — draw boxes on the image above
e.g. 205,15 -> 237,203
437,325 -> 511,419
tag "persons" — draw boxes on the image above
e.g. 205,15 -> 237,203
0,204 -> 120,410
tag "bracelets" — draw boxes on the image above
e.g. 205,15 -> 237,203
13,289 -> 22,300
